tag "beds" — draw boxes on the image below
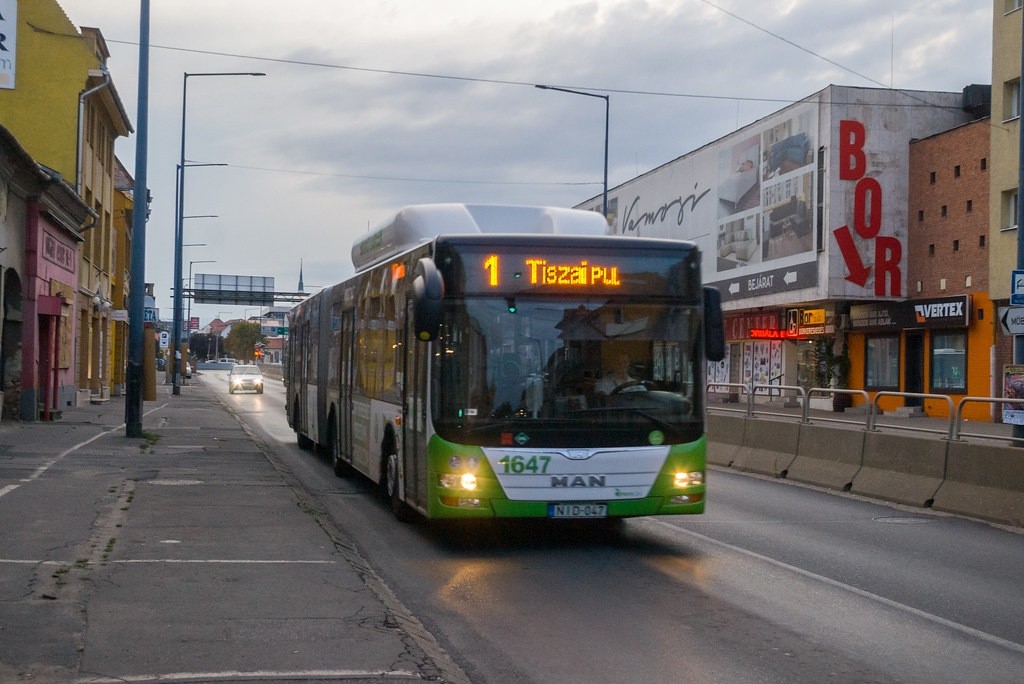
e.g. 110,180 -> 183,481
718,145 -> 757,209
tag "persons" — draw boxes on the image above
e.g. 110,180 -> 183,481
596,350 -> 649,395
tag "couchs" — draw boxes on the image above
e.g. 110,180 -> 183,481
771,132 -> 808,168
722,231 -> 757,260
767,195 -> 809,238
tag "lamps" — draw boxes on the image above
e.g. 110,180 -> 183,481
102,301 -> 112,307
92,296 -> 100,305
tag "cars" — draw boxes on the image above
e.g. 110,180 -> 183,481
227,364 -> 266,394
206,360 -> 217,363
160,359 -> 165,366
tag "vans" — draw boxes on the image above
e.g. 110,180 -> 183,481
216,357 -> 239,365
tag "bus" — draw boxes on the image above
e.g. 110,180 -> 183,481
283,203 -> 725,522
186,361 -> 194,379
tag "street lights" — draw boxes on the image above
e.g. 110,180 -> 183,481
174,163 -> 229,259
172,70 -> 267,395
187,260 -> 219,358
243,307 -> 260,321
537,85 -> 610,219
